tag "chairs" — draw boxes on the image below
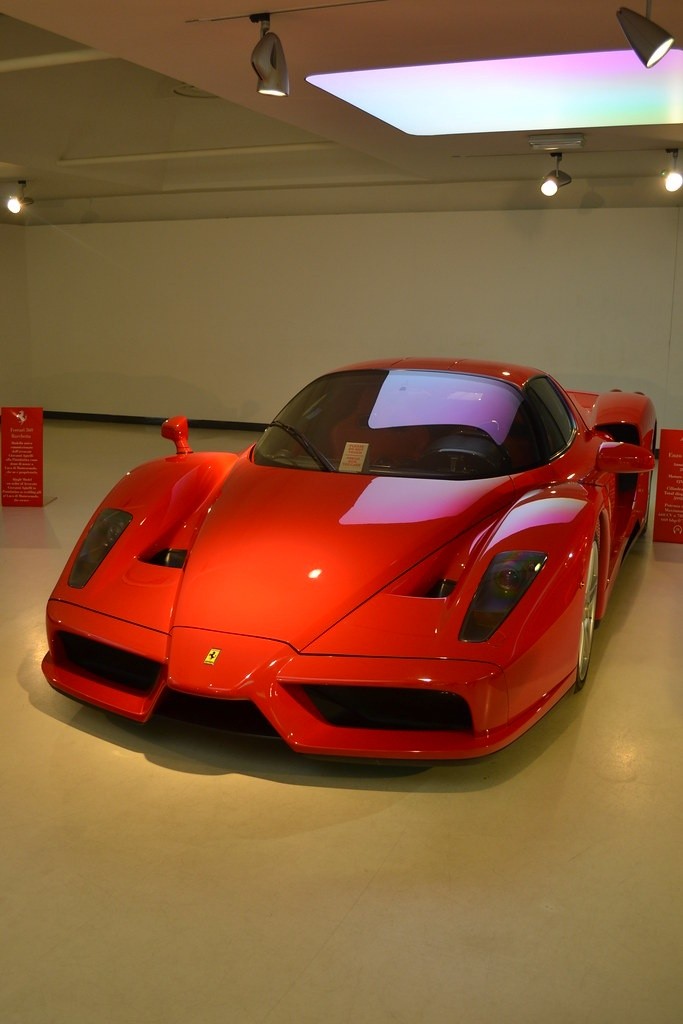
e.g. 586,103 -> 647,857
331,373 -> 429,469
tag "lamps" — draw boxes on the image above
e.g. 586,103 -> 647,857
249,12 -> 290,97
7,180 -> 34,213
661,148 -> 683,191
541,153 -> 571,196
617,0 -> 675,69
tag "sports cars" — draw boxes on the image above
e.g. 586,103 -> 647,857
35,353 -> 659,766
